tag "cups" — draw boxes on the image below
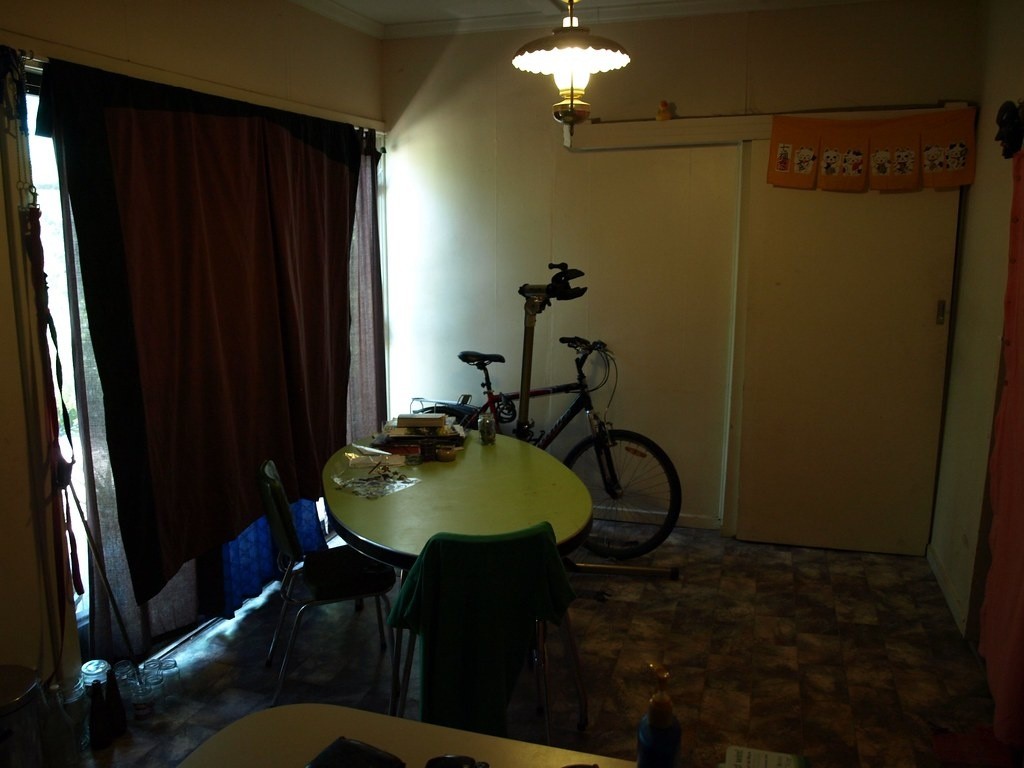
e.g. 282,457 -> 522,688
436,439 -> 455,462
418,437 -> 437,462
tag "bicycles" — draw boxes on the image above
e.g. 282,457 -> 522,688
408,334 -> 682,561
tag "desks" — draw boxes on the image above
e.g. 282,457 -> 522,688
321,428 -> 595,591
174,702 -> 640,768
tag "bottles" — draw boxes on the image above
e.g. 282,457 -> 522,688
88,669 -> 127,751
113,658 -> 180,720
0,663 -> 45,768
44,684 -> 78,768
478,412 -> 496,446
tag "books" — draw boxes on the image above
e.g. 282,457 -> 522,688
397,413 -> 446,428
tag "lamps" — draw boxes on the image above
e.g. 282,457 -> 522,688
511,0 -> 632,137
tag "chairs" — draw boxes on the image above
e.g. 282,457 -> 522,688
260,457 -> 398,681
386,520 -> 586,747
